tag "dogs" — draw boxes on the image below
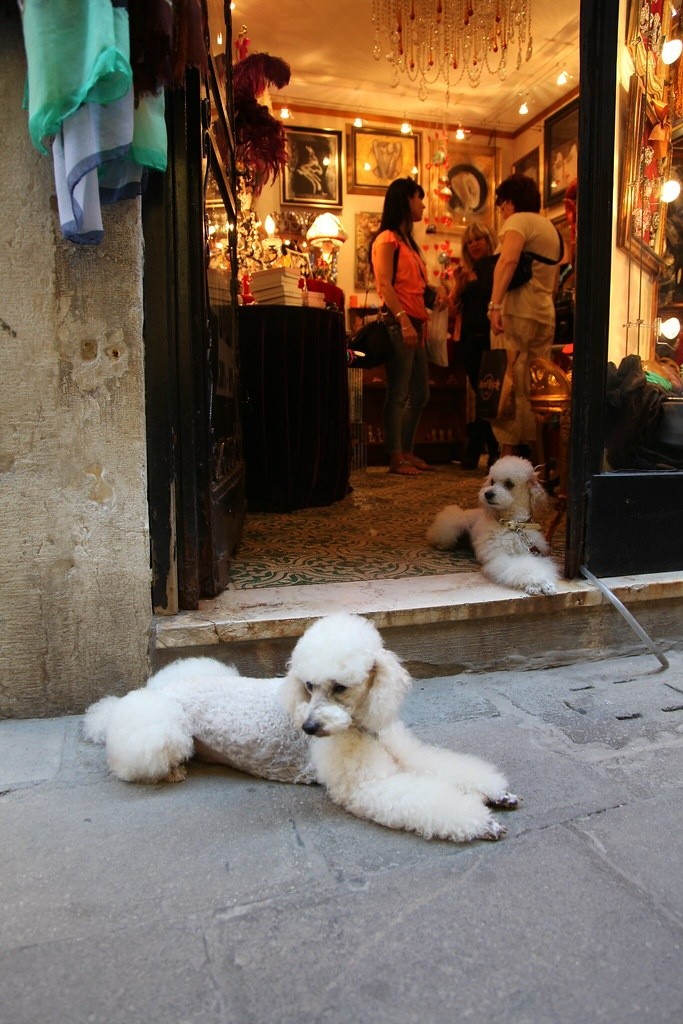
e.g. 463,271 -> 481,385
79,612 -> 520,842
424,456 -> 560,597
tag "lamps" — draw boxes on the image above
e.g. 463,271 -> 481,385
305,213 -> 349,284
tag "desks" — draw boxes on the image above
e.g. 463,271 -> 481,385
238,304 -> 352,514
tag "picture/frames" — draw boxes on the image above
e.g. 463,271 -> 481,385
346,122 -> 423,196
616,72 -> 673,271
541,93 -> 580,223
279,124 -> 345,209
428,139 -> 502,239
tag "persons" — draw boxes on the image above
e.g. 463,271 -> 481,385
369,179 -> 441,475
447,221 -> 500,470
488,174 -> 560,483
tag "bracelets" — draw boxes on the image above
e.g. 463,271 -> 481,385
400,324 -> 412,330
395,310 -> 405,317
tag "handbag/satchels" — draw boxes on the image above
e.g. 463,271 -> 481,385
474,248 -> 534,293
348,320 -> 394,370
423,288 -> 437,310
465,333 -> 507,421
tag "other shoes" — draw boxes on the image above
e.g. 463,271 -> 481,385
487,452 -> 500,466
459,441 -> 484,470
389,456 -> 436,475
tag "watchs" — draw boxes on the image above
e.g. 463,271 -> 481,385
488,302 -> 502,310
